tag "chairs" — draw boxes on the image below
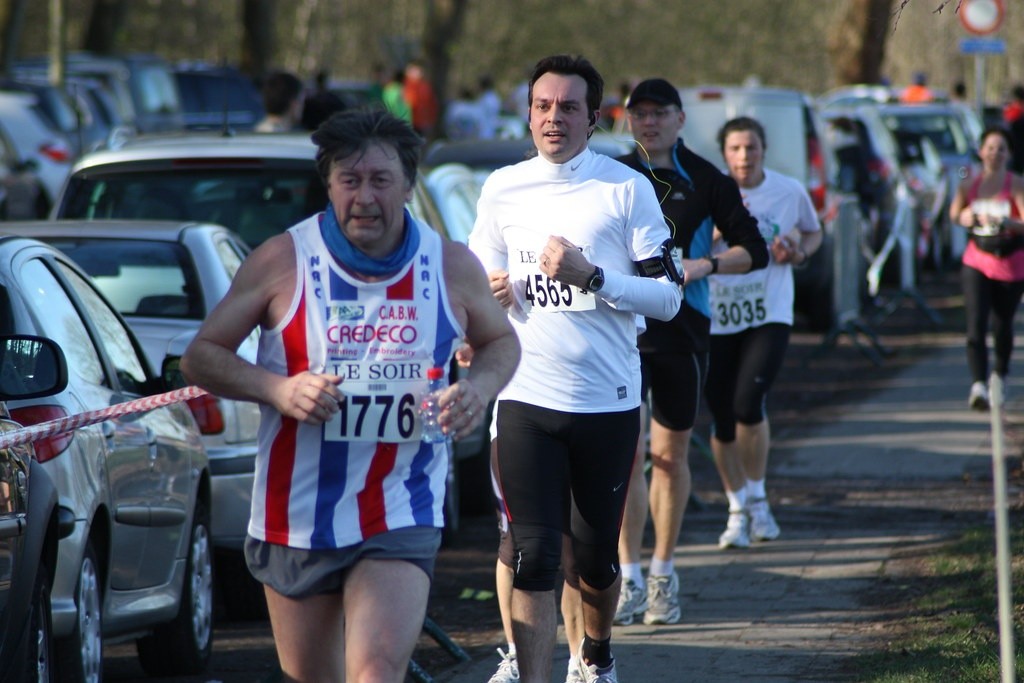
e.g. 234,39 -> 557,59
136,294 -> 188,315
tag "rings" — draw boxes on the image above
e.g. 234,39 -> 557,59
466,410 -> 472,416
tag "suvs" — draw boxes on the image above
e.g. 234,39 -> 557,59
48,122 -> 467,551
135,55 -> 265,136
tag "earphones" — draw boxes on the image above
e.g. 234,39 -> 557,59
589,113 -> 596,126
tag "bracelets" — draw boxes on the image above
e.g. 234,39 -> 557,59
799,249 -> 807,266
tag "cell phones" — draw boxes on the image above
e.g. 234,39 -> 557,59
667,240 -> 684,284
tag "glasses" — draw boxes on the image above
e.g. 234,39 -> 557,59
629,106 -> 678,120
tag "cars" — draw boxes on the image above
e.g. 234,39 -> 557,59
1,216 -> 265,683
805,89 -> 990,295
0,49 -> 135,216
423,133 -> 630,171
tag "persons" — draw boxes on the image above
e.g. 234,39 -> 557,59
900,72 -> 933,102
700,117 -> 824,548
1001,85 -> 1024,175
611,79 -> 769,625
178,106 -> 520,683
381,62 -> 439,135
453,54 -> 684,683
300,70 -> 343,125
251,73 -> 304,132
948,84 -> 967,103
949,128 -> 1024,412
447,74 -> 502,115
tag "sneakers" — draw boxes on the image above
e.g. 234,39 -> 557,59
614,577 -> 650,623
488,646 -> 521,683
717,511 -> 750,548
967,379 -> 990,408
746,496 -> 780,542
565,655 -> 587,683
642,571 -> 681,625
988,371 -> 1005,408
577,637 -> 617,683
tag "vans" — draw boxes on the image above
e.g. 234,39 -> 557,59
627,80 -> 831,329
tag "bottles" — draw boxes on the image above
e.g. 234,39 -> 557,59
420,366 -> 451,443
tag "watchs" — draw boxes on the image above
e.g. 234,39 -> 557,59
704,254 -> 718,274
584,266 -> 605,291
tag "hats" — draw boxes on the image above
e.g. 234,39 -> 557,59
626,79 -> 682,109
